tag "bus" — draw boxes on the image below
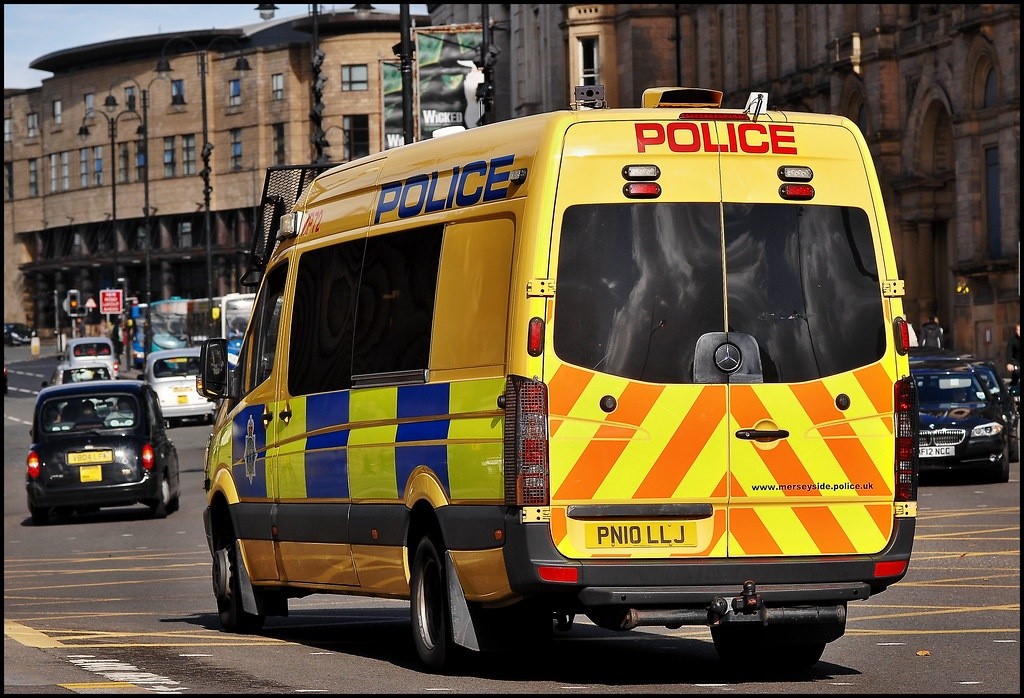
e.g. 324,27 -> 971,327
126,294 -> 192,373
185,291 -> 283,372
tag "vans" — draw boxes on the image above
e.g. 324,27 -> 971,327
55,337 -> 119,377
197,85 -> 918,669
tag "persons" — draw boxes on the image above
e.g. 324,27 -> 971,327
920,314 -> 944,348
111,319 -> 124,365
1004,323 -> 1020,387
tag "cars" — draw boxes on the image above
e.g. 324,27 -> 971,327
906,344 -> 1022,482
137,346 -> 219,425
4,323 -> 38,346
24,379 -> 181,524
39,361 -> 121,414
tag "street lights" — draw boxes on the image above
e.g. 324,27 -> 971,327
151,34 -> 254,340
254,0 -> 379,180
72,106 -> 147,288
102,72 -> 189,351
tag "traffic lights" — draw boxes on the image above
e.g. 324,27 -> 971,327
78,305 -> 87,317
68,290 -> 80,318
